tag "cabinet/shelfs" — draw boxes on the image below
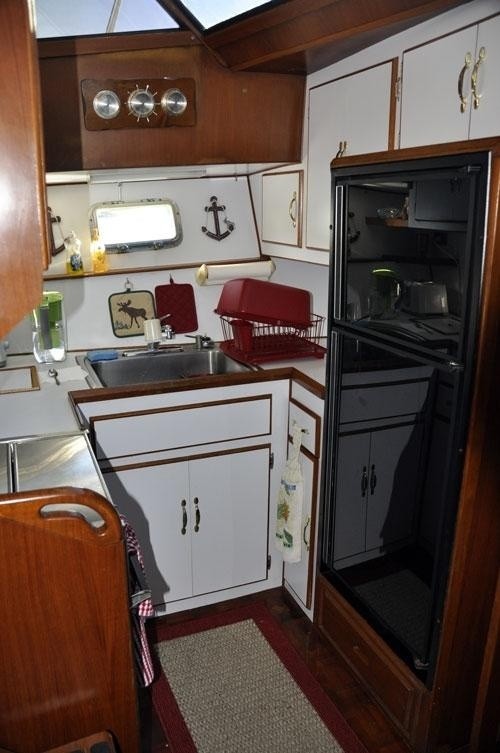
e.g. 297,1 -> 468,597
314,132 -> 500,753
75,380 -> 278,605
305,59 -> 395,256
397,12 -> 500,151
283,377 -> 318,607
333,365 -> 438,570
259,169 -> 304,246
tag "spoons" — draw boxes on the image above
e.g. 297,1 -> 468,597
49,368 -> 60,385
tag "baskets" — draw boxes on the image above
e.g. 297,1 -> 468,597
218,311 -> 323,353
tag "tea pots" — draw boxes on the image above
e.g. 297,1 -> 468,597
370,268 -> 406,320
30,290 -> 67,363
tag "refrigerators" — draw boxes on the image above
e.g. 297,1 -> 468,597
321,154 -> 493,688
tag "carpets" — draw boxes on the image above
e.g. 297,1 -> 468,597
147,602 -> 371,753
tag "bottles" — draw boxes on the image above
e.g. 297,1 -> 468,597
64,227 -> 108,275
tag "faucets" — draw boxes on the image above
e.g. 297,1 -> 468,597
147,341 -> 160,352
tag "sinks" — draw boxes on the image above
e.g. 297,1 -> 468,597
75,344 -> 265,388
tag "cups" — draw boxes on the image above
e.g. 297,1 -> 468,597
144,319 -> 163,345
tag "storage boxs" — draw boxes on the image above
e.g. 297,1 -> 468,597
214,276 -> 328,365
